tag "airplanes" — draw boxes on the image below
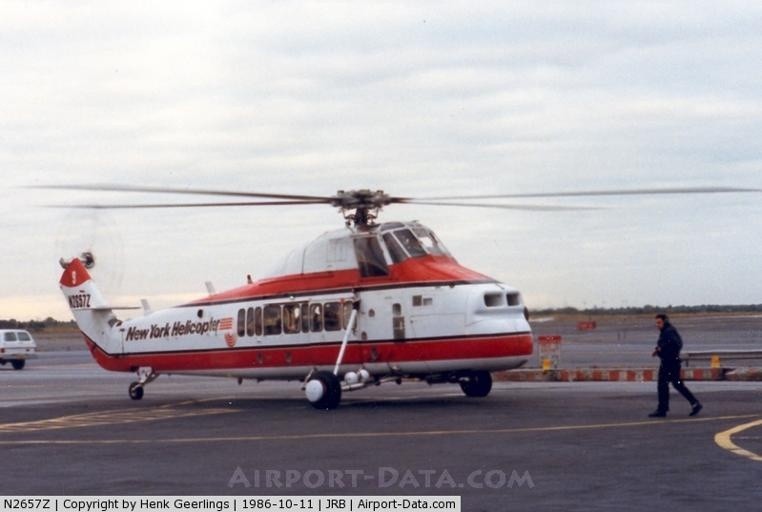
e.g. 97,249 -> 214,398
12,182 -> 762,413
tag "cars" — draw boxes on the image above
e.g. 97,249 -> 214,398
0,329 -> 37,370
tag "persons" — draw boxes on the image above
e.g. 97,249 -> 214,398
644,312 -> 703,418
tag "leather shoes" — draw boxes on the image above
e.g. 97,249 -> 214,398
648,411 -> 666,417
689,405 -> 702,416
